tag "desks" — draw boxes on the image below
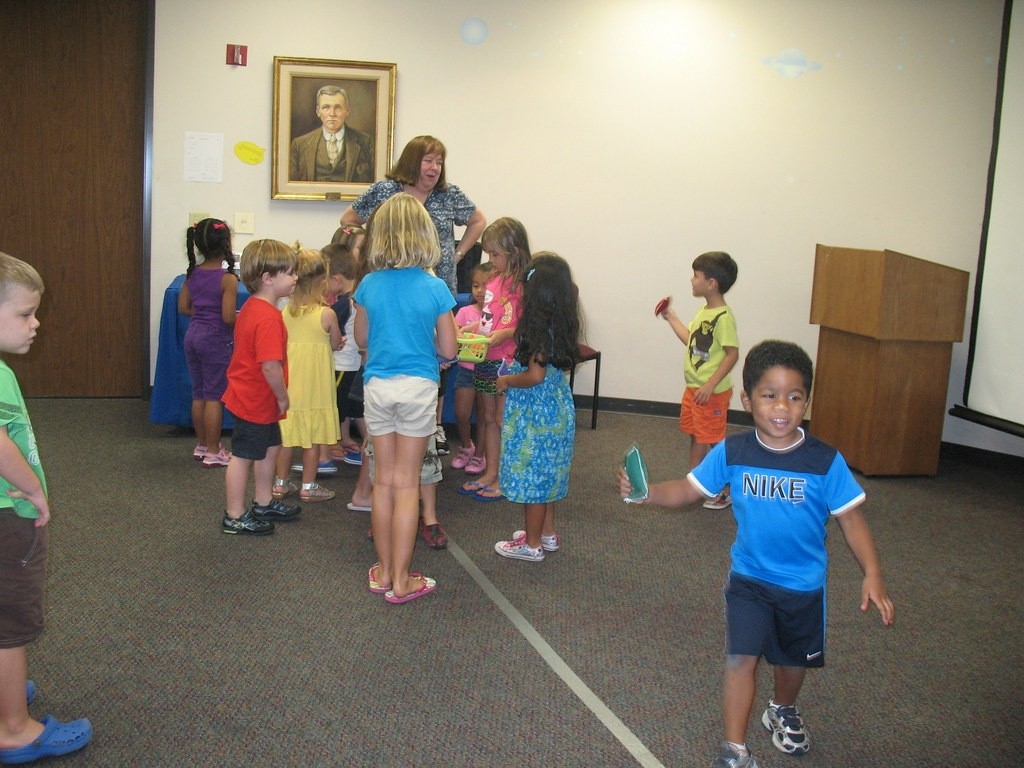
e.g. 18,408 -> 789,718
146,269 -> 476,426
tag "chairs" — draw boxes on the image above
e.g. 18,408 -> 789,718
569,343 -> 600,429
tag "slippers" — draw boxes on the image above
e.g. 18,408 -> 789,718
329,441 -> 363,461
383,572 -> 437,604
368,561 -> 395,595
456,479 -> 509,502
26,679 -> 37,707
346,502 -> 373,511
0,712 -> 94,765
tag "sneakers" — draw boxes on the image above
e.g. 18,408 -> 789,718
494,538 -> 545,562
707,741 -> 759,768
223,509 -> 275,537
434,426 -> 452,456
251,496 -> 303,521
193,442 -> 233,469
511,529 -> 560,552
451,438 -> 476,469
464,455 -> 488,475
761,697 -> 811,756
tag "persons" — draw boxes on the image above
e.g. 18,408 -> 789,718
616,339 -> 893,768
455,217 -> 530,501
0,251 -> 92,762
221,240 -> 302,534
494,254 -> 580,560
341,135 -> 486,453
273,194 -> 466,604
180,218 -> 240,467
662,252 -> 740,509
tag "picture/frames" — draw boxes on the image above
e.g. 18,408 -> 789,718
271,57 -> 397,202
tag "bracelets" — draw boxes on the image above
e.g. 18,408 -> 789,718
455,248 -> 465,257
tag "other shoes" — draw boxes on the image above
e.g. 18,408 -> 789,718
367,526 -> 374,541
289,459 -> 339,475
423,522 -> 448,549
701,491 -> 732,509
343,451 -> 364,466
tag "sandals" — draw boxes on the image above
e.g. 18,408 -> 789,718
270,476 -> 298,500
299,480 -> 336,502
